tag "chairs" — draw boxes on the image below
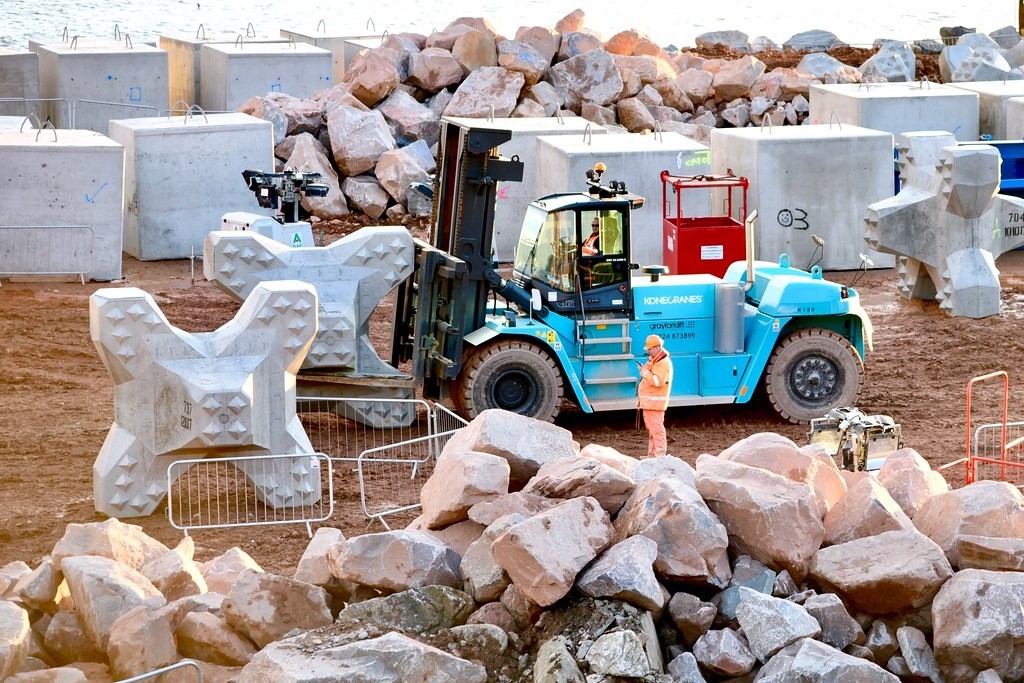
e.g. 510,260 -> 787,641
581,216 -> 619,284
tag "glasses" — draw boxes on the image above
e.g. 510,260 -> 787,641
592,224 -> 599,227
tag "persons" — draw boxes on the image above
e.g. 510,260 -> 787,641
636,335 -> 673,459
559,217 -> 606,291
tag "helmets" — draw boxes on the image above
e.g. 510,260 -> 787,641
644,335 -> 662,350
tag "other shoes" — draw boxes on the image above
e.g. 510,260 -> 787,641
639,456 -> 648,460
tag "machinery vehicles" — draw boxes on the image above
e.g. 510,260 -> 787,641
294,123 -> 871,424
